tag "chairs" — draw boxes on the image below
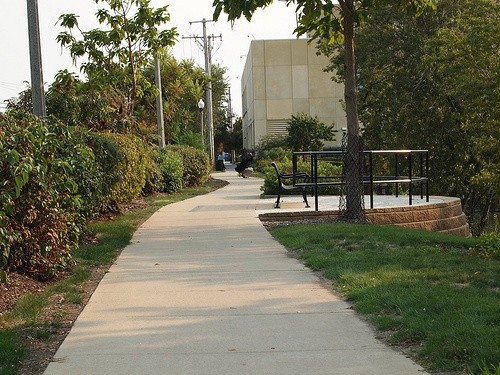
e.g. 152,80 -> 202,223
270,161 -> 312,209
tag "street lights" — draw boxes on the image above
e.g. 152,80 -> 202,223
197,98 -> 205,153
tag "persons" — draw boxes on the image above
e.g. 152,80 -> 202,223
234,148 -> 254,177
217,150 -> 227,171
